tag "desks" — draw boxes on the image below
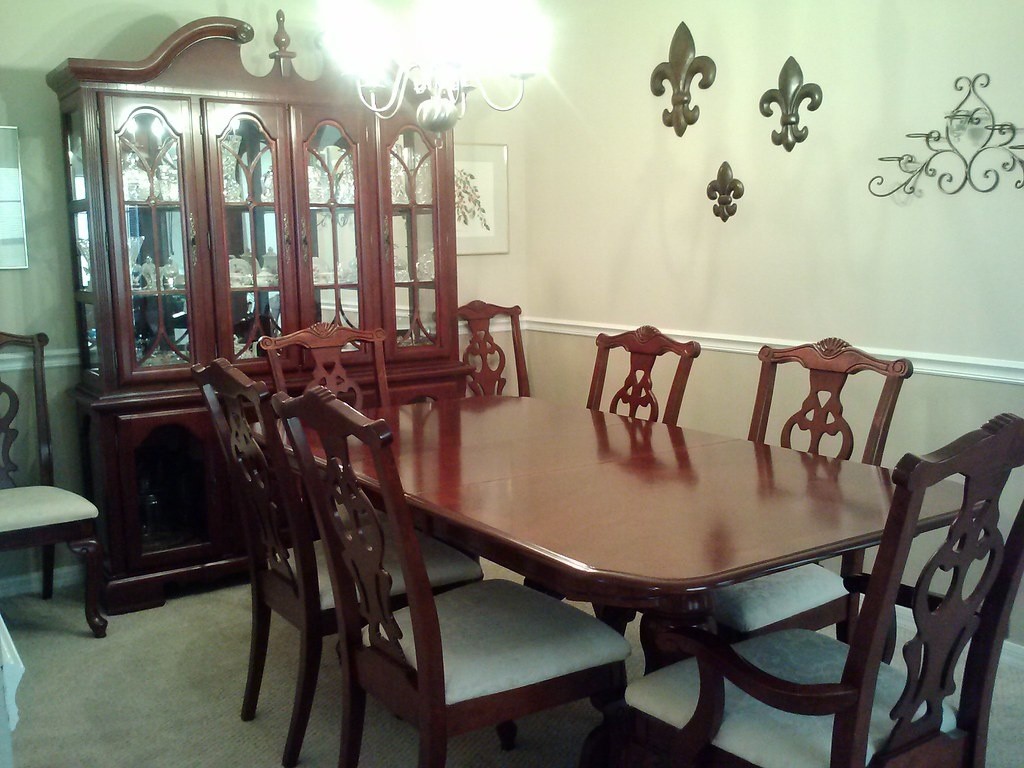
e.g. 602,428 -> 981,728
248,396 -> 987,768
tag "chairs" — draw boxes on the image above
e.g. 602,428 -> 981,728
605,414 -> 1024,768
527,326 -> 703,626
258,323 -> 390,415
272,384 -> 632,768
705,338 -> 914,647
0,332 -> 110,640
191,357 -> 484,768
454,300 -> 530,398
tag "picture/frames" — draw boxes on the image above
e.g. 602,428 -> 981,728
452,144 -> 511,257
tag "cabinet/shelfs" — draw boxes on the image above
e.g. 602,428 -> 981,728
44,9 -> 478,616
